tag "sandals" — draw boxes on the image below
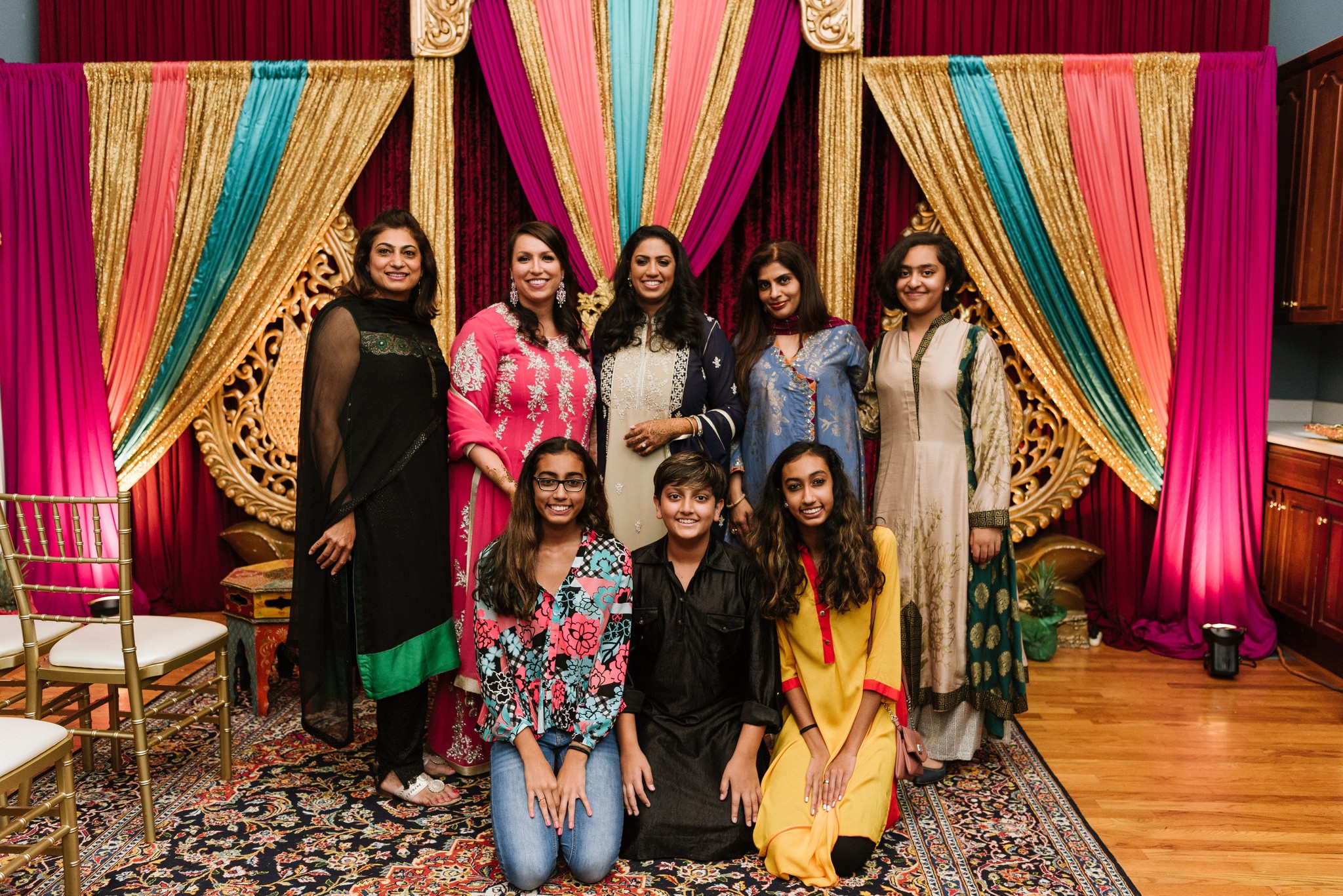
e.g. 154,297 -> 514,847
418,749 -> 457,783
377,764 -> 467,809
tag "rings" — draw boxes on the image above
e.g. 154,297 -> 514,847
537,797 -> 546,802
822,780 -> 830,784
730,529 -> 737,535
642,442 -> 647,449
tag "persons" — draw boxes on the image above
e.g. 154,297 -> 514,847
287,208 -> 469,811
583,223 -> 743,560
614,452 -> 784,863
725,238 -> 872,542
855,231 -> 1031,787
740,440 -> 905,888
426,217 -> 603,776
471,436 -> 639,892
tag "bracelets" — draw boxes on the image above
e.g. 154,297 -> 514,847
799,723 -> 818,735
567,743 -> 591,759
726,492 -> 746,509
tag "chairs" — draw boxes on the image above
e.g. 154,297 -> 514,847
0,490 -> 235,844
0,615 -> 95,771
0,718 -> 81,896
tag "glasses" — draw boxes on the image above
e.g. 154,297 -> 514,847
533,475 -> 590,494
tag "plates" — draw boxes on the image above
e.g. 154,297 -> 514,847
1304,422 -> 1343,442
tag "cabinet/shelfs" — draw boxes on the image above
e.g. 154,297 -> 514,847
1272,34 -> 1343,323
1258,421 -> 1343,643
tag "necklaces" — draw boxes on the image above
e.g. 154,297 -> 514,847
684,414 -> 703,438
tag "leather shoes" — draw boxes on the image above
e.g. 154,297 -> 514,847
912,754 -> 947,786
